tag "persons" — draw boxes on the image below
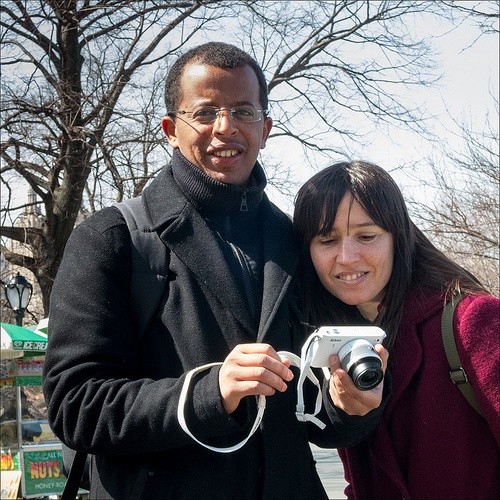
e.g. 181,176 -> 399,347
293,161 -> 500,500
42,41 -> 389,500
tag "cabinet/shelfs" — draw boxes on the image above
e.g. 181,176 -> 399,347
0,359 -> 64,500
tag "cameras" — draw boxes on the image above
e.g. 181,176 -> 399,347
306,326 -> 387,391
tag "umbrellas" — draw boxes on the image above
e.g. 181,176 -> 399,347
0,322 -> 47,360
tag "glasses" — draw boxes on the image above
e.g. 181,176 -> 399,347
165,105 -> 270,123
